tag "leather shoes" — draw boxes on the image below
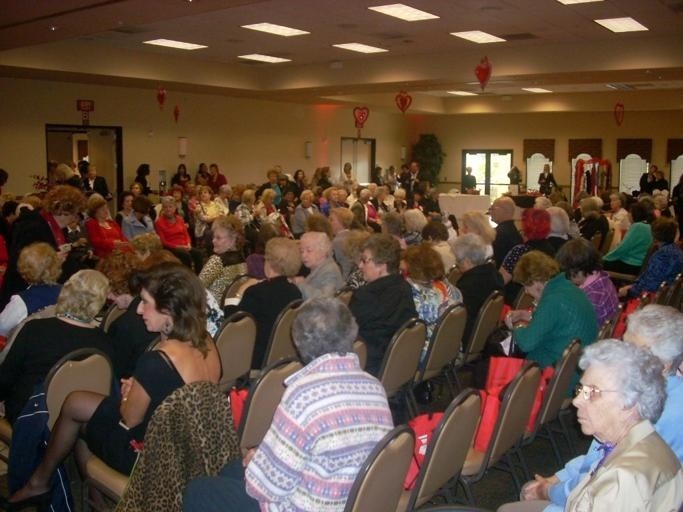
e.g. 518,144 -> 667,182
416,380 -> 433,404
1,489 -> 53,511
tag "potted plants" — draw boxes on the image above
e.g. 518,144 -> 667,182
507,166 -> 522,195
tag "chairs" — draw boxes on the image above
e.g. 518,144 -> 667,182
1,210 -> 370,511
495,337 -> 582,500
459,361 -> 542,511
370,211 -> 595,419
595,210 -> 682,342
342,425 -> 417,511
398,386 -> 482,512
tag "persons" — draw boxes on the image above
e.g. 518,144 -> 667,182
0,160 -> 682,511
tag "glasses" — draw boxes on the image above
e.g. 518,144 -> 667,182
575,384 -> 617,400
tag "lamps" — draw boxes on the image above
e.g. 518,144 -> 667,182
175,135 -> 189,157
304,140 -> 315,157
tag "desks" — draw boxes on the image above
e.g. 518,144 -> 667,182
438,191 -> 491,214
502,191 -> 545,207
